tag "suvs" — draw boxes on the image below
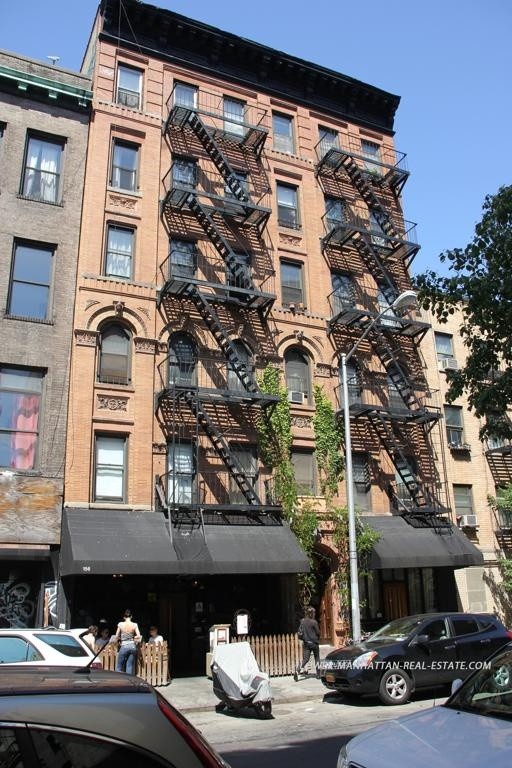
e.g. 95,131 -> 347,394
317,612 -> 511,705
1,628 -> 102,671
0,641 -> 231,768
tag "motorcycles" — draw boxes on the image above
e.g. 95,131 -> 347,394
210,640 -> 273,720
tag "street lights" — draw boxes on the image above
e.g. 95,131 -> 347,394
340,289 -> 418,644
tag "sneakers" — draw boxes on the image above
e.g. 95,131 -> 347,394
294,672 -> 299,682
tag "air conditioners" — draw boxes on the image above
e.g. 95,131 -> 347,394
442,358 -> 457,369
287,390 -> 305,403
459,513 -> 477,527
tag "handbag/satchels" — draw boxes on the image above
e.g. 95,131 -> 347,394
296,617 -> 306,641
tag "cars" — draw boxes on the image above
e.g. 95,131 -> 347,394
336,641 -> 511,767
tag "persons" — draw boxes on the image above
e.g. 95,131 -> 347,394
82,624 -> 100,651
96,628 -> 110,650
148,626 -> 163,652
294,607 -> 321,682
115,609 -> 140,676
